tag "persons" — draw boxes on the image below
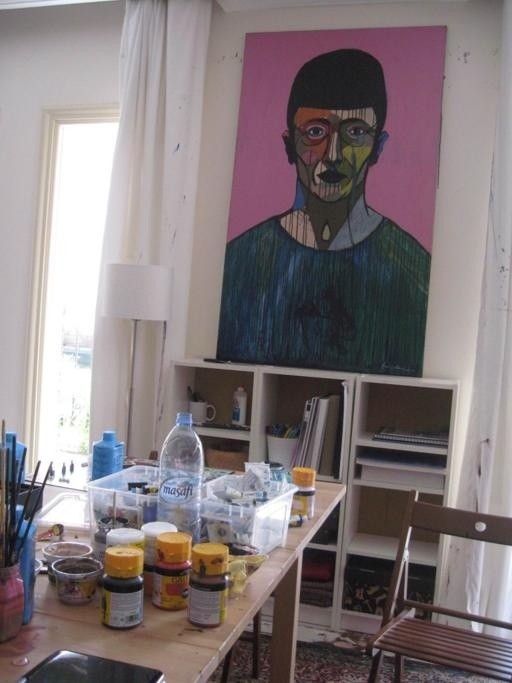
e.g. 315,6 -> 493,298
214,42 -> 437,375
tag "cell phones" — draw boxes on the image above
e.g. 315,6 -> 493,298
18,649 -> 165,683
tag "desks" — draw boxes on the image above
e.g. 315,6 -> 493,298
0,469 -> 347,683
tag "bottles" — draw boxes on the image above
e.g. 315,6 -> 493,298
187,542 -> 229,629
91,430 -> 125,482
105,521 -> 193,611
231,387 -> 247,426
98,544 -> 145,630
156,411 -> 205,547
290,467 -> 316,520
1,431 -> 27,483
7,504 -> 37,626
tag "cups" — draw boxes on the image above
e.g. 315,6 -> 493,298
50,556 -> 104,607
189,400 -> 216,426
42,542 -> 93,587
266,435 -> 298,471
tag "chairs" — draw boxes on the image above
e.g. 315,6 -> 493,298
367,487 -> 512,683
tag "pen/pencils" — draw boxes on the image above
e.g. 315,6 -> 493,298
290,512 -> 309,528
187,386 -> 197,400
203,359 -> 227,363
0,420 -> 53,568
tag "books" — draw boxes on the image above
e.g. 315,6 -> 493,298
288,391 -> 340,479
356,426 -> 450,493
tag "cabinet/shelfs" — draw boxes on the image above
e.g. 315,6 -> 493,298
162,358 -> 461,634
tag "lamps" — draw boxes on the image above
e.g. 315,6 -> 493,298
98,262 -> 173,457
